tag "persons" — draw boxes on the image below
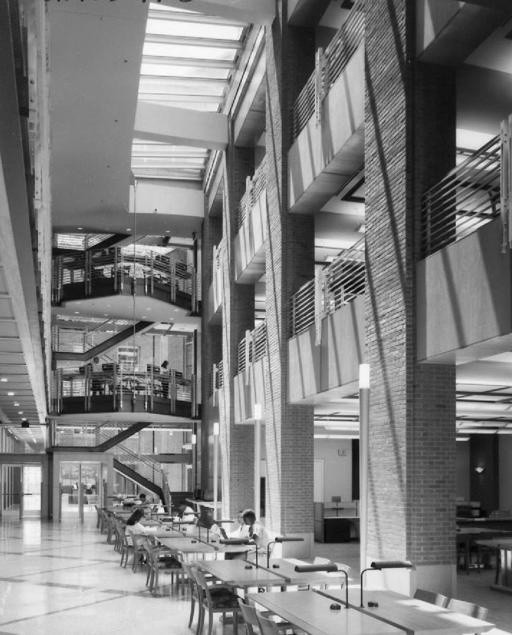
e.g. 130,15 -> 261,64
172,501 -> 194,530
90,357 -> 105,396
224,508 -> 250,561
132,494 -> 152,511
124,509 -> 160,564
231,510 -> 264,560
160,360 -> 171,399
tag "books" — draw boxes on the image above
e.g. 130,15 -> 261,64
219,526 -> 228,540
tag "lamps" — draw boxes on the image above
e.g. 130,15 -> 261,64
331,495 -> 341,517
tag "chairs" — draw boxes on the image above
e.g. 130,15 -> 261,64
94,505 -> 511,635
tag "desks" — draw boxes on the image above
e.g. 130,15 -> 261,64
185,497 -> 221,526
312,502 -> 359,544
